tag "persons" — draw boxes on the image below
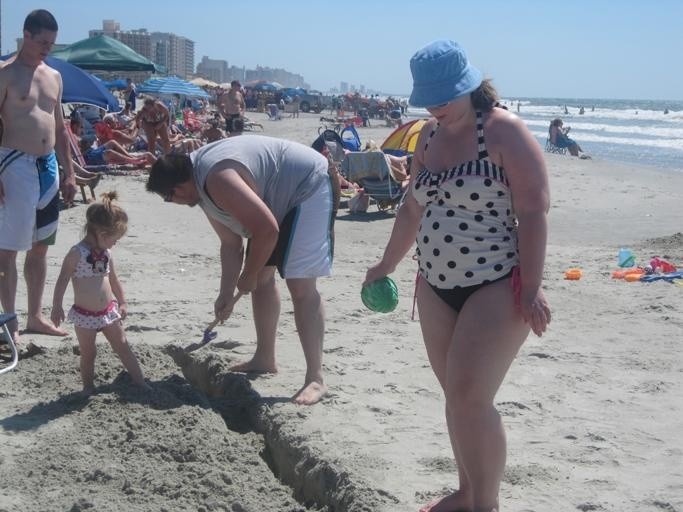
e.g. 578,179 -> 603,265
504,99 -> 520,112
57,75 -> 246,165
51,191 -> 153,397
146,135 -> 340,405
332,91 -> 408,127
246,87 -> 300,118
362,40 -> 551,512
548,104 -> 595,157
0,10 -> 77,346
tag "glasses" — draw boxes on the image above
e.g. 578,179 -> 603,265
164,191 -> 173,202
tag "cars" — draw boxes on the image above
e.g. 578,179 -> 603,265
244,82 -> 325,113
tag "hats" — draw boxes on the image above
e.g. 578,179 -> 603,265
408,40 -> 483,108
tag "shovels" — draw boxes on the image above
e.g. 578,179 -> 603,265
183,291 -> 244,354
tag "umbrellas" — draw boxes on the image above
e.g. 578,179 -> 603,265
0,49 -> 125,112
49,34 -> 156,74
